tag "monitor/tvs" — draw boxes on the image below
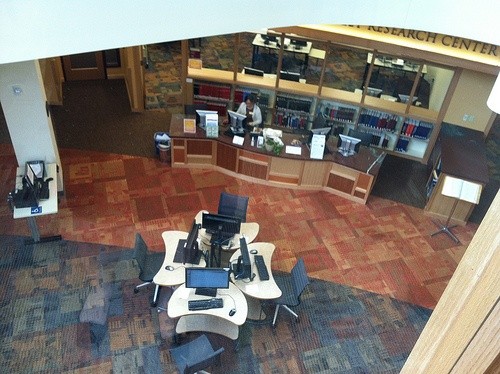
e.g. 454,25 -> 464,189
194,110 -> 218,127
280,71 -> 300,82
261,34 -> 276,45
232,238 -> 253,281
397,94 -> 419,107
244,67 -> 264,76
184,267 -> 229,296
202,212 -> 241,244
307,127 -> 333,146
337,134 -> 362,157
226,109 -> 248,134
185,224 -> 203,264
23,176 -> 38,206
362,86 -> 383,98
290,39 -> 307,49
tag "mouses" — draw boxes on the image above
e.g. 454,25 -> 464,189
250,249 -> 258,254
198,224 -> 201,229
46,177 -> 54,182
165,265 -> 174,271
229,309 -> 236,316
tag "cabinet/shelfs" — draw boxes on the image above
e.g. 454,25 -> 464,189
424,122 -> 489,225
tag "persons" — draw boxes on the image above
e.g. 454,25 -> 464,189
223,93 -> 262,129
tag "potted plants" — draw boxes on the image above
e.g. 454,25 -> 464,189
263,137 -> 282,155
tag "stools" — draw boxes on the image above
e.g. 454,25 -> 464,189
309,48 -> 326,72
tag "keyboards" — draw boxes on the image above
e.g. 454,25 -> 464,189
173,239 -> 186,262
254,255 -> 269,281
188,298 -> 223,310
38,181 -> 49,201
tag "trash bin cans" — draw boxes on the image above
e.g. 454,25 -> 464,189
152,132 -> 171,159
158,144 -> 171,163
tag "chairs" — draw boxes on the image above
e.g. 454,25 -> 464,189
132,233 -> 166,307
269,258 -> 310,327
169,333 -> 225,374
219,192 -> 249,221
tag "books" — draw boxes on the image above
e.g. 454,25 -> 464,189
192,82 -> 269,123
272,95 -> 434,153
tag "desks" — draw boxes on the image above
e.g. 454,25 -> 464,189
187,66 -> 440,164
251,34 -> 312,77
166,283 -> 248,341
12,165 -> 62,245
354,88 -> 422,107
152,230 -> 207,287
228,242 -> 282,322
359,53 -> 427,97
194,211 -> 260,251
241,68 -> 306,84
